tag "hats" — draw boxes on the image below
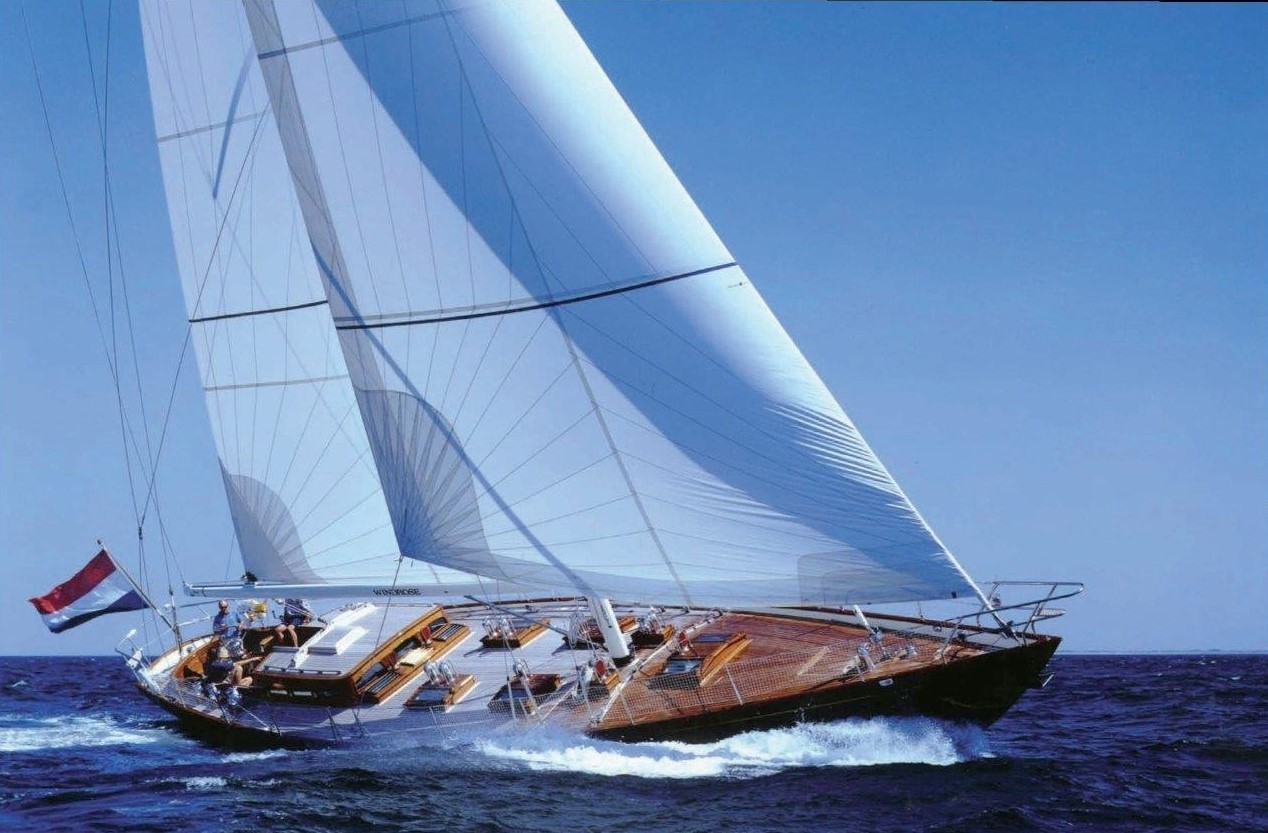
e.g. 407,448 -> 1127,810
217,647 -> 229,657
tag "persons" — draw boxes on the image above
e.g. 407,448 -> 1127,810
204,648 -> 253,689
237,602 -> 266,636
276,596 -> 310,648
213,600 -> 244,661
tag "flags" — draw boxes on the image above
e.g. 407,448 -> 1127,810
28,549 -> 151,634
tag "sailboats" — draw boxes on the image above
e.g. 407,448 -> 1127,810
19,1 -> 1086,754
